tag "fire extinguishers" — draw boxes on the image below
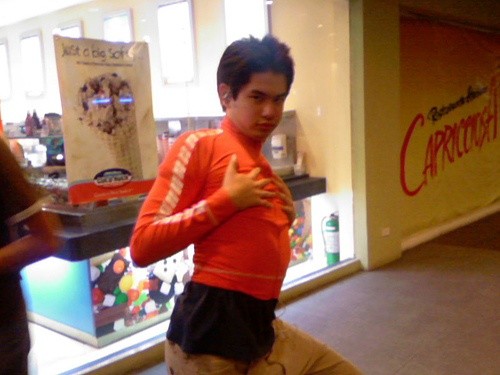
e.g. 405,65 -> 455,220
321,210 -> 340,266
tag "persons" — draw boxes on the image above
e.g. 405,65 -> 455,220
131,34 -> 364,374
0,137 -> 58,375
